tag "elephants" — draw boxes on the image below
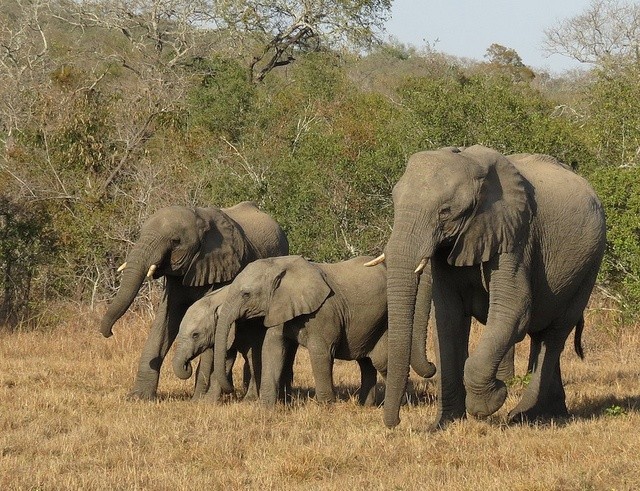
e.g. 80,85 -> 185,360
97,198 -> 411,408
363,142 -> 608,433
